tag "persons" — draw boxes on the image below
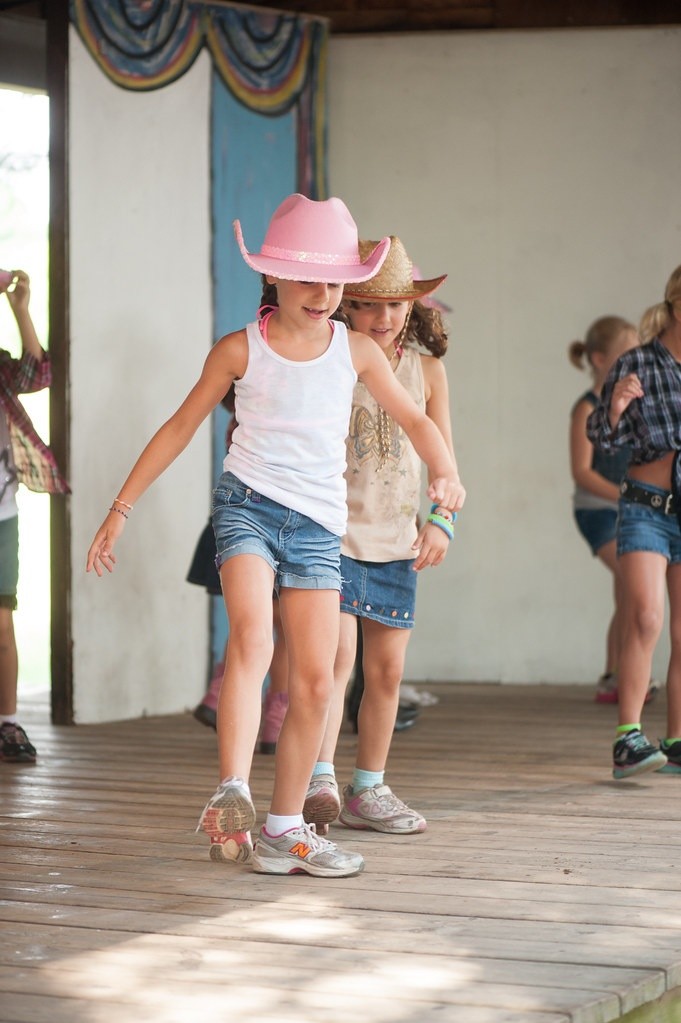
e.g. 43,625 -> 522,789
568,314 -> 662,707
84,191 -> 466,880
585,261 -> 681,779
0,265 -> 73,761
302,232 -> 466,844
182,376 -> 306,754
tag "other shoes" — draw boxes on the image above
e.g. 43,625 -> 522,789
0,721 -> 36,761
595,671 -> 656,705
350,701 -> 419,737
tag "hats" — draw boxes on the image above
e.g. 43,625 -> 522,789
234,193 -> 393,283
343,238 -> 446,302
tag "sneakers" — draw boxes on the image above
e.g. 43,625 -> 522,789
656,737 -> 681,773
339,779 -> 426,835
195,775 -> 256,865
611,727 -> 668,778
301,773 -> 342,835
251,822 -> 364,878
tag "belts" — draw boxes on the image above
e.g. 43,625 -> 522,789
620,479 -> 677,516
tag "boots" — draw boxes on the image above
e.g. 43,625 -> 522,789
259,688 -> 290,754
193,661 -> 225,731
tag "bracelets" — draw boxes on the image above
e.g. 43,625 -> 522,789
426,503 -> 457,540
113,498 -> 134,511
110,507 -> 128,520
